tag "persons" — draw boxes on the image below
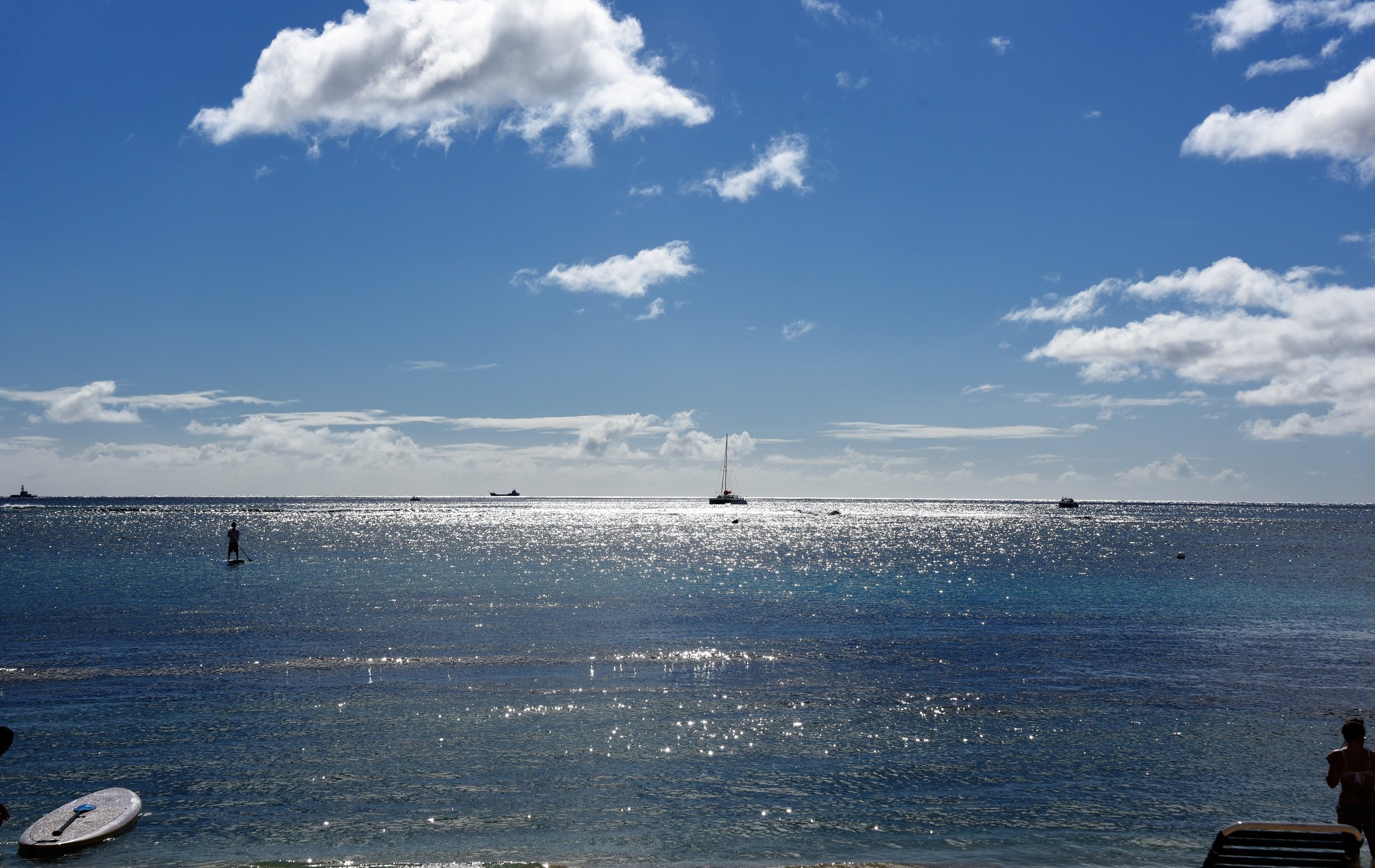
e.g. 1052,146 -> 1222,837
1324,717 -> 1375,868
227,521 -> 240,560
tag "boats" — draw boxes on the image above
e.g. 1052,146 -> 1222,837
10,485 -> 38,497
1058,496 -> 1079,508
489,489 -> 520,496
410,496 -> 420,501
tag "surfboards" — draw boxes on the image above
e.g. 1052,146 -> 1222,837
19,788 -> 142,850
225,559 -> 244,563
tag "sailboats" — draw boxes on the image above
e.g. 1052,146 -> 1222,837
709,434 -> 748,505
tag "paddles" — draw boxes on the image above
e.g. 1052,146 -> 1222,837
230,532 -> 252,561
52,804 -> 95,835
